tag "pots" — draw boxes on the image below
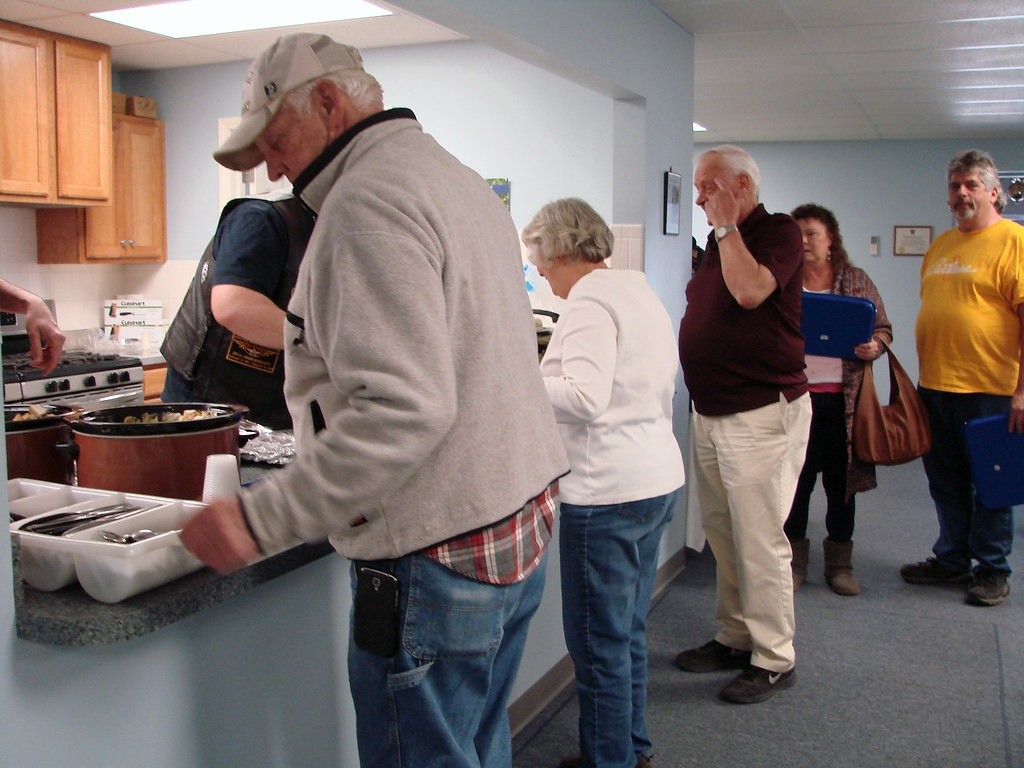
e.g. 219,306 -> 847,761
63,402 -> 250,499
3,403 -> 82,487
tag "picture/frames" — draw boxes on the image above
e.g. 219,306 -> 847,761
663,171 -> 681,237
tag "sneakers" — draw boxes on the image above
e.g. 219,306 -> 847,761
966,567 -> 1010,606
718,661 -> 797,704
901,557 -> 973,586
675,638 -> 753,674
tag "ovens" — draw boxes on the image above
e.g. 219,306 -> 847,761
16,383 -> 144,407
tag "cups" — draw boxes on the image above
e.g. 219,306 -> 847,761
201,455 -> 241,503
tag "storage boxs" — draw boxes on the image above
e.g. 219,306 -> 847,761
126,96 -> 157,119
112,91 -> 127,114
103,294 -> 169,356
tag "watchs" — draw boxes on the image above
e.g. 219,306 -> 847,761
715,225 -> 738,244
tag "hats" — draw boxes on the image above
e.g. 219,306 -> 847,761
213,32 -> 363,172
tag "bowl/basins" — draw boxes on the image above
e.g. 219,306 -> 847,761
238,429 -> 259,449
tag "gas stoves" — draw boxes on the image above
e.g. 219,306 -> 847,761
0,298 -> 144,402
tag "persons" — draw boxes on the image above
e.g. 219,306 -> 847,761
522,197 -> 686,768
783,203 -> 893,594
179,33 -> 571,768
692,236 -> 705,276
0,279 -> 66,376
159,186 -> 318,431
675,144 -> 813,703
900,150 -> 1024,605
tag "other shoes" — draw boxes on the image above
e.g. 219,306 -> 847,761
560,745 -> 652,768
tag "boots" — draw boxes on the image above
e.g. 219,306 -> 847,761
823,535 -> 861,596
789,538 -> 810,593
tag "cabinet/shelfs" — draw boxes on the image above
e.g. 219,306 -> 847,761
35,114 -> 168,264
0,18 -> 113,208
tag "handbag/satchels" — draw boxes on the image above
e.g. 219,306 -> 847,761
852,332 -> 934,465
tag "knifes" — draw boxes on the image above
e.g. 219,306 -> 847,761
18,502 -> 148,538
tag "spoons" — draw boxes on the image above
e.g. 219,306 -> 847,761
94,528 -> 166,544
